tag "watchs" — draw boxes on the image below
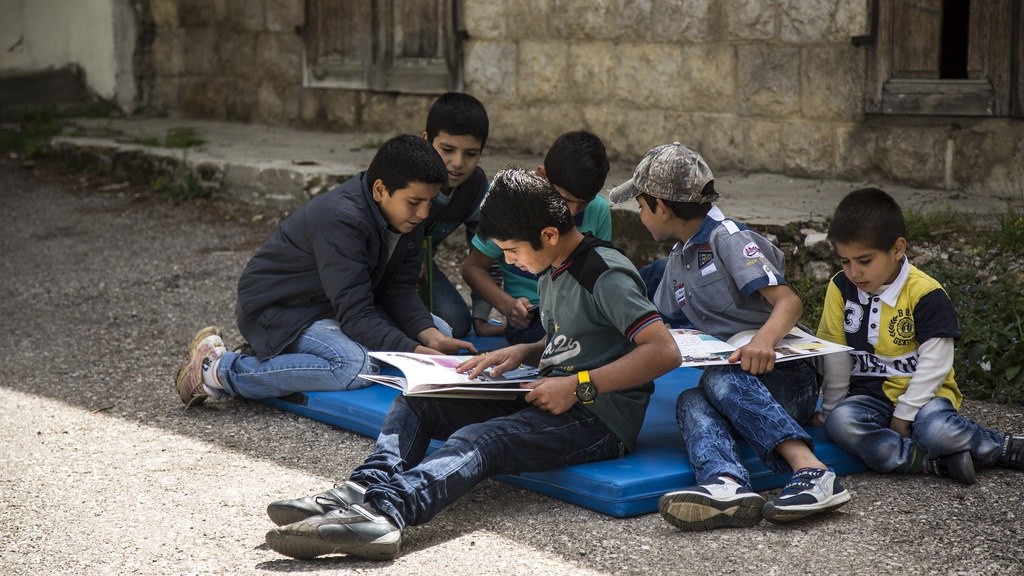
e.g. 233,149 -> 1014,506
575,370 -> 597,405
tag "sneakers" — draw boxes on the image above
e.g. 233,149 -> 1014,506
177,326 -> 225,411
926,451 -> 975,485
266,501 -> 400,559
266,478 -> 368,527
996,433 -> 1024,469
763,467 -> 851,523
658,476 -> 766,533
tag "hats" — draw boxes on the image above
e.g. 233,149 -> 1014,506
608,141 -> 721,205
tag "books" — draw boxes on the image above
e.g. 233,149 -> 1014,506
357,349 -> 545,401
662,326 -> 854,366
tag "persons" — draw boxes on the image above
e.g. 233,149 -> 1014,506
460,132 -> 692,367
608,142 -> 852,534
266,163 -> 682,561
811,188 -> 1024,485
175,136 -> 478,408
421,92 -> 506,339
